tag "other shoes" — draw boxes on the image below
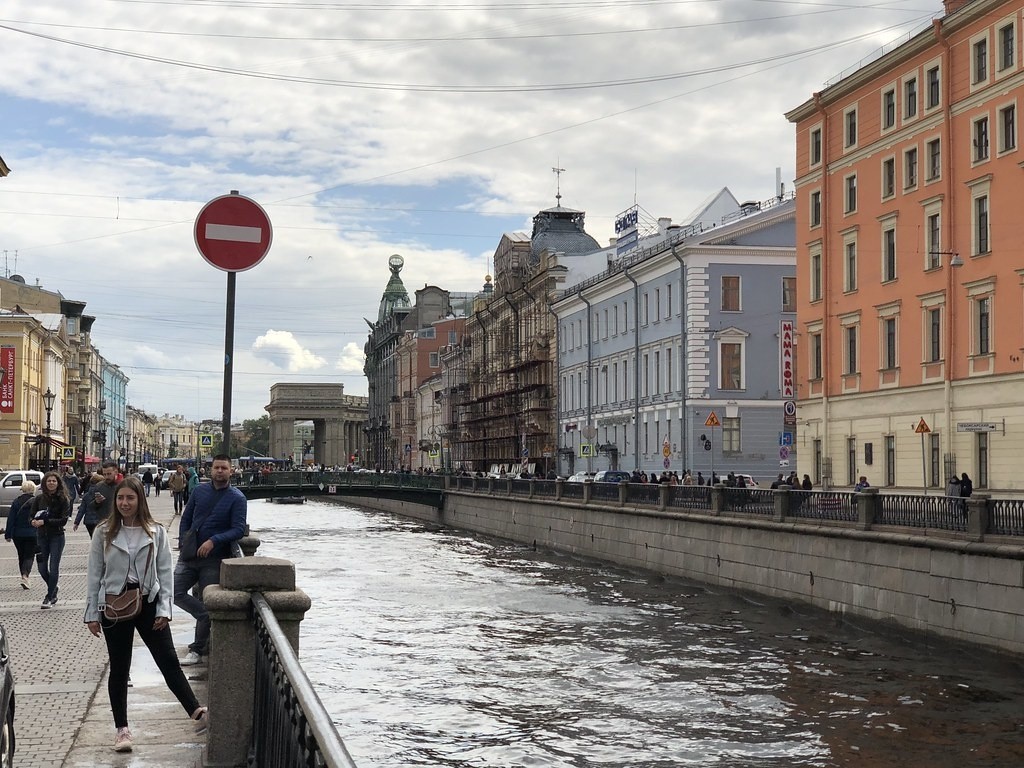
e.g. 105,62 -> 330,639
21,578 -> 28,589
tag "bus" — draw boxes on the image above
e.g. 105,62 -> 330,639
128,440 -> 292,490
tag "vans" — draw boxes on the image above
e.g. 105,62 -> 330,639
0,470 -> 45,517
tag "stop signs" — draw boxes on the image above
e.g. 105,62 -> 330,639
194,195 -> 273,272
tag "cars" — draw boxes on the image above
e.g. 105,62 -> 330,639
0,624 -> 15,768
566,471 -> 630,496
719,474 -> 760,501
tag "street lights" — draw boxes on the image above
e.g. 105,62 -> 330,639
41,388 -> 56,472
80,406 -> 162,476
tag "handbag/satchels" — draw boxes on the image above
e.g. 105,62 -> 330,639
182,530 -> 197,559
104,588 -> 141,619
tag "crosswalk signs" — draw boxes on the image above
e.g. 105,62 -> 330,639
62,446 -> 76,460
200,434 -> 213,447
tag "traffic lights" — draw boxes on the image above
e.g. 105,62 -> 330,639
351,455 -> 355,460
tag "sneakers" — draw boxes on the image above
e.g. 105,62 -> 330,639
115,726 -> 132,751
41,596 -> 52,609
192,706 -> 209,736
52,587 -> 58,604
178,652 -> 201,664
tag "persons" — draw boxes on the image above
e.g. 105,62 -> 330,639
62,464 -> 124,540
626,470 -> 747,509
169,463 -> 206,515
770,471 -> 813,503
84,477 -> 207,751
520,470 -> 557,480
456,465 -> 483,477
402,465 -> 433,475
142,468 -> 153,497
28,471 -> 69,608
154,473 -> 162,497
855,476 -> 870,492
171,454 -> 247,666
4,481 -> 37,589
947,473 -> 972,519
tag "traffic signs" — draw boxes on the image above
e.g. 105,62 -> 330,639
957,422 -> 996,432
